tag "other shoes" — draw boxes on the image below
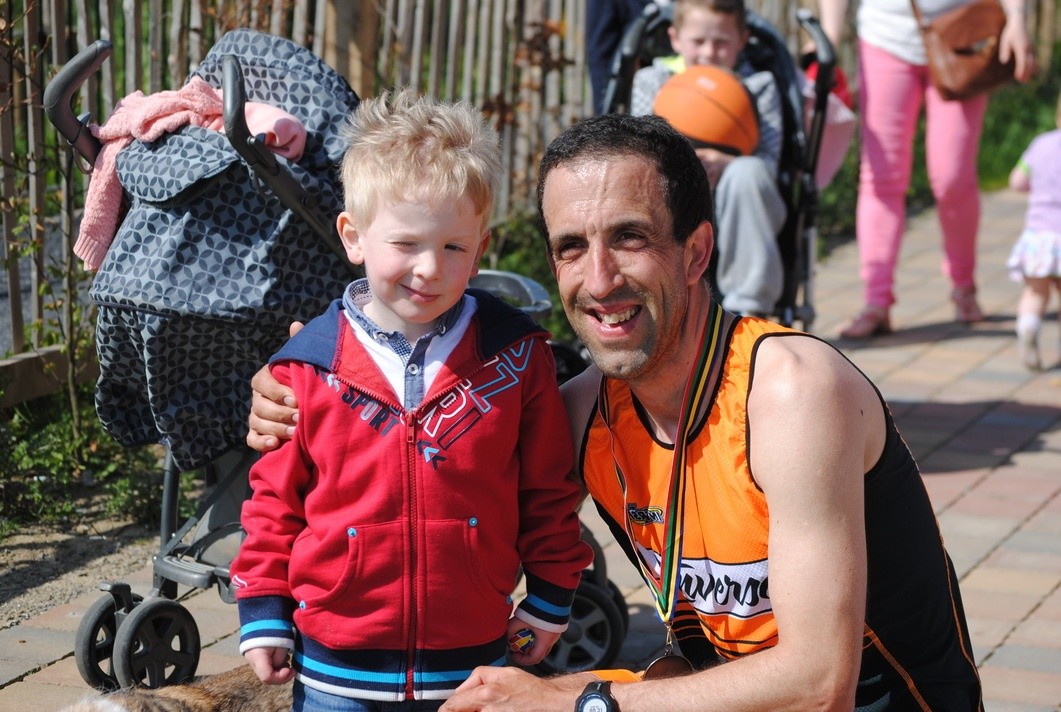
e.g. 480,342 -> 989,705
949,285 -> 982,329
839,304 -> 890,340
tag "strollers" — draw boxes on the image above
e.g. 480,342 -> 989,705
44,27 -> 627,691
605,0 -> 839,334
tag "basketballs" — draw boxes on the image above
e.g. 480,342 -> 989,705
653,64 -> 762,157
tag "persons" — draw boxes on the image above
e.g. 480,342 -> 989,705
1006,90 -> 1061,372
229,84 -> 594,712
244,112 -> 989,712
809,2 -> 1036,345
625,1 -> 789,326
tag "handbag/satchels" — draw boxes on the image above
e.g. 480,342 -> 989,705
911,1 -> 1017,102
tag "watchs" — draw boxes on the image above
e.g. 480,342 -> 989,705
573,678 -> 620,712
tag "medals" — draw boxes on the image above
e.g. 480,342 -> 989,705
642,650 -> 694,682
